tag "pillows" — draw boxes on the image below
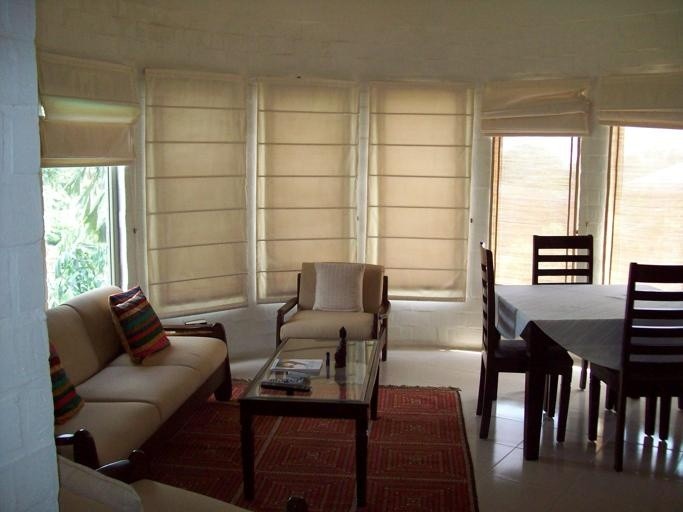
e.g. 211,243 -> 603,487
311,261 -> 365,312
41,285 -> 171,424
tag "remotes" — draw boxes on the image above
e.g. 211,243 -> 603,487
260,377 -> 312,392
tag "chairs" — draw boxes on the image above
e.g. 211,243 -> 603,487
276,263 -> 390,362
48,286 -> 307,512
475,241 -> 574,461
531,236 -> 594,286
584,261 -> 682,472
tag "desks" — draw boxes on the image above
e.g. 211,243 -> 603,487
495,282 -> 682,449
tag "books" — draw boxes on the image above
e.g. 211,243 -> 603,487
184,318 -> 207,325
270,357 -> 324,373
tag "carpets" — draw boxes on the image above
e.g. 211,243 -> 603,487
125,380 -> 480,512
